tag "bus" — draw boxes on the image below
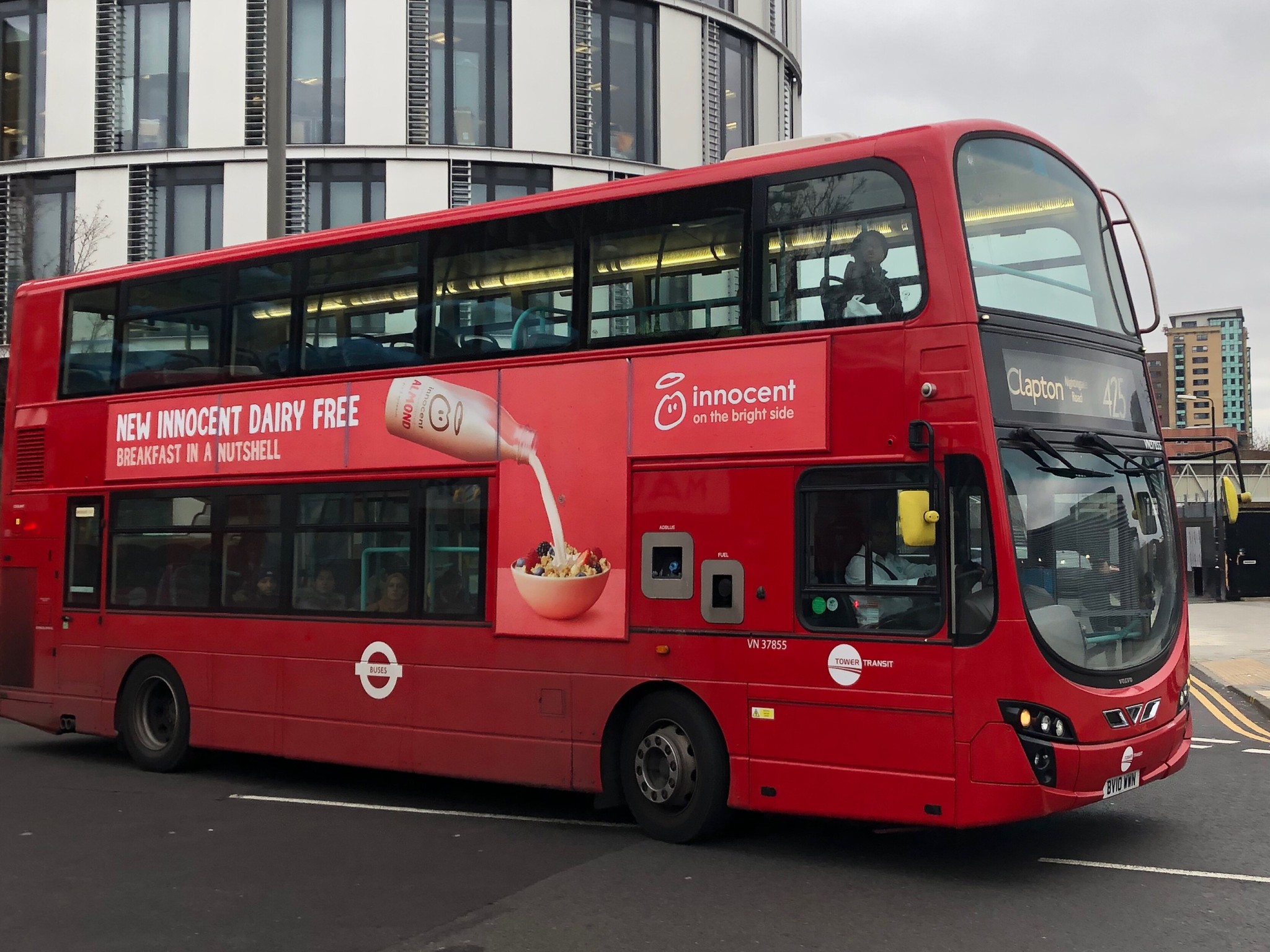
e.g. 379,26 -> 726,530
1,117 -> 1253,840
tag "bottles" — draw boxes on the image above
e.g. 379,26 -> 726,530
384,376 -> 538,467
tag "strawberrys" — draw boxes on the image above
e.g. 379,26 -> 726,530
578,546 -> 602,565
525,549 -> 541,573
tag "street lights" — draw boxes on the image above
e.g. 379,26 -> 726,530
1176,394 -> 1224,603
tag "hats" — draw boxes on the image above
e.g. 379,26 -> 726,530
851,231 -> 888,263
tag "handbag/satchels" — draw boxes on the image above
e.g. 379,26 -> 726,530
845,293 -> 882,318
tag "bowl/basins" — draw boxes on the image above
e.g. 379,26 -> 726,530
510,560 -> 614,619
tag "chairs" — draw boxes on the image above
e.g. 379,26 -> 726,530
818,274 -> 848,323
111,559 -> 366,616
64,326 -> 503,394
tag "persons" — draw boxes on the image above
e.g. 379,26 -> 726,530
827,231 -> 904,319
1080,554 -> 1119,667
846,495 -> 979,629
71,527 -> 467,612
